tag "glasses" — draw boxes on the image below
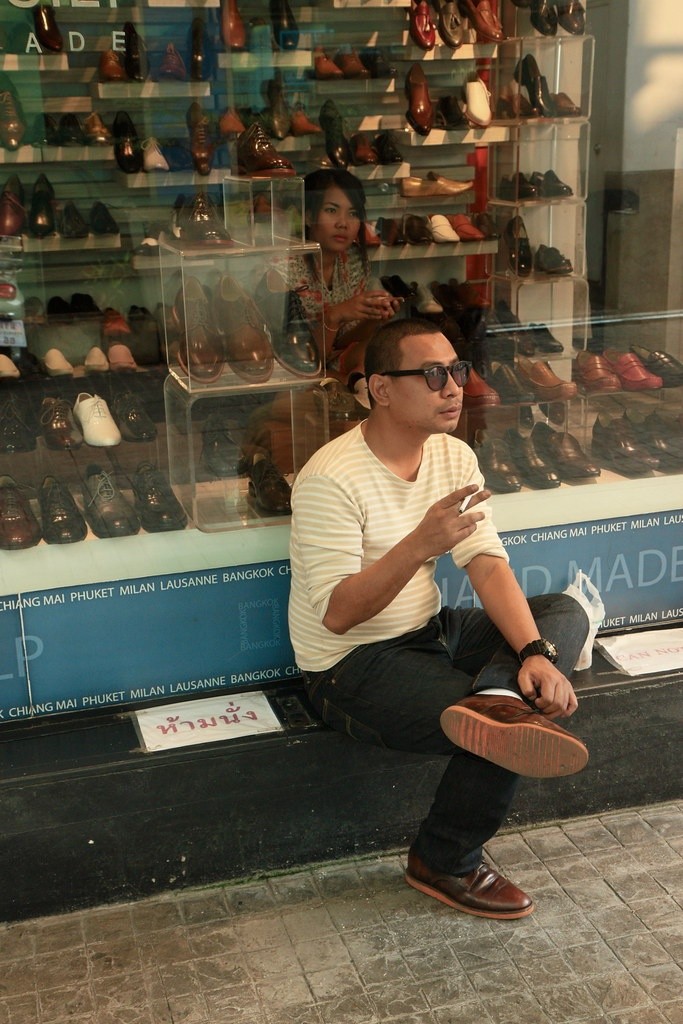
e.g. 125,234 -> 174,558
379,359 -> 474,391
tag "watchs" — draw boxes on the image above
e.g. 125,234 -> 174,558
519,637 -> 559,665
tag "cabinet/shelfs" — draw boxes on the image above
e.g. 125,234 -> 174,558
1,0 -> 597,483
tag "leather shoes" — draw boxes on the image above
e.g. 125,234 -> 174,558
405,846 -> 534,920
440,695 -> 588,777
0,0 -> 683,550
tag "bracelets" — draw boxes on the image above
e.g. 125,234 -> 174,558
324,322 -> 341,332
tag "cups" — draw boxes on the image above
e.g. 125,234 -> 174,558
574,609 -> 606,671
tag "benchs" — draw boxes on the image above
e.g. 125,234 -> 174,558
0,623 -> 683,910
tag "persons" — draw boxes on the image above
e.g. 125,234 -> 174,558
289,316 -> 590,920
244,167 -> 402,370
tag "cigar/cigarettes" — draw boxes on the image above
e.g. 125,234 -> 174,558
459,494 -> 472,514
